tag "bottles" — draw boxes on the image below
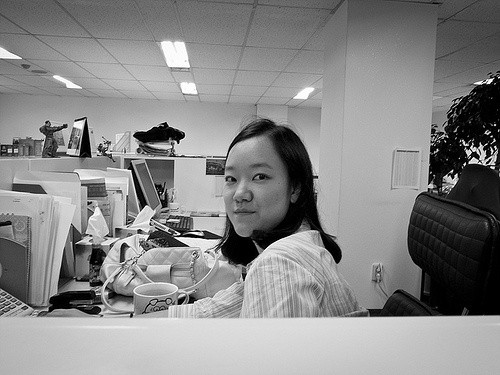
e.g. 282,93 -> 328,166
88,244 -> 106,287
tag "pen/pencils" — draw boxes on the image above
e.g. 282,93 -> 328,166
155,179 -> 167,200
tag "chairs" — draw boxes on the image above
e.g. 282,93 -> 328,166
376,191 -> 500,315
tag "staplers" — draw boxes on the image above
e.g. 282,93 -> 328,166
47,290 -> 102,315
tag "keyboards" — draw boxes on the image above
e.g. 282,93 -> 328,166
0,288 -> 35,318
166,214 -> 193,231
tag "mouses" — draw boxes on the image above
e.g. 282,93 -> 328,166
182,229 -> 204,237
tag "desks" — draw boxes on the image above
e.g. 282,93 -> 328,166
28,212 -> 227,321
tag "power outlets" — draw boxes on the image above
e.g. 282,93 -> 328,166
371,263 -> 384,282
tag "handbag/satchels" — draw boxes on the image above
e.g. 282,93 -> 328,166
99,233 -> 243,313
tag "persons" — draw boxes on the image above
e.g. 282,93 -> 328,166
39,120 -> 68,157
45,114 -> 369,318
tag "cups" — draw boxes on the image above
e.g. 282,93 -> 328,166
133,282 -> 189,315
169,203 -> 181,215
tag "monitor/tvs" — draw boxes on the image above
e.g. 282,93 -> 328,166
130,159 -> 163,214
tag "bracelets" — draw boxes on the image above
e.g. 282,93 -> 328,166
99,314 -> 103,318
